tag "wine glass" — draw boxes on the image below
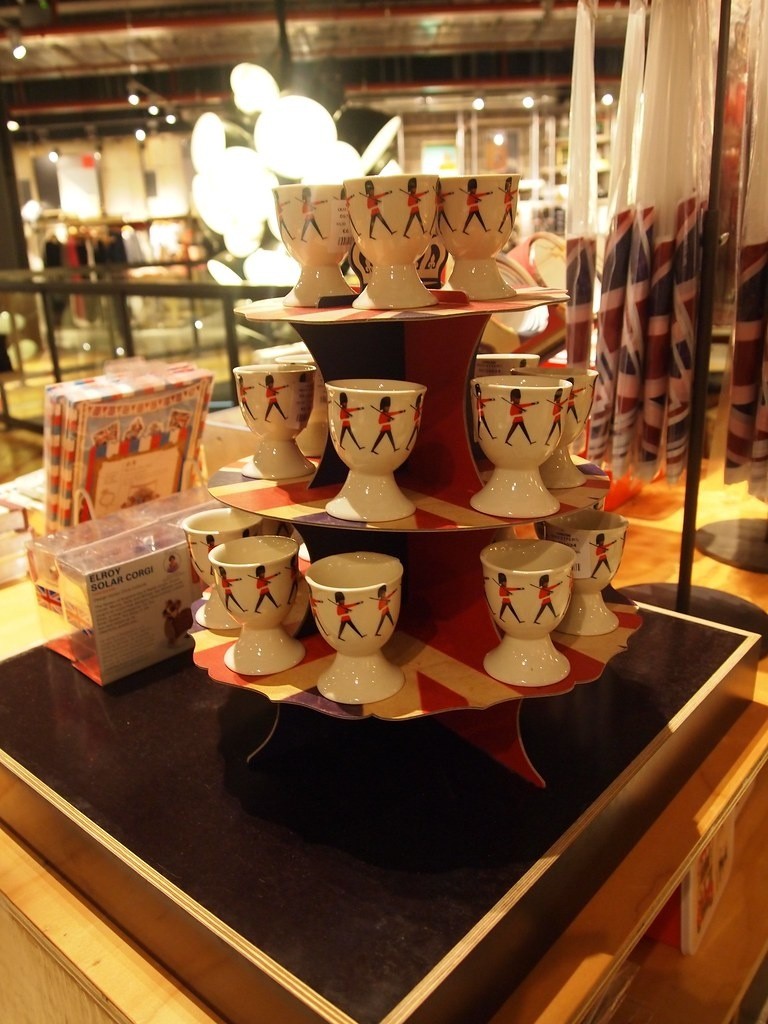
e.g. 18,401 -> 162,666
479,511 -> 629,689
467,352 -> 601,520
269,172 -> 524,311
180,507 -> 408,705
233,349 -> 428,524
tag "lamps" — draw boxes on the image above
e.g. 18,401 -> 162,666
190,61 -> 407,288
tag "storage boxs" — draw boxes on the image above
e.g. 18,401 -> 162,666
29,492 -> 216,687
